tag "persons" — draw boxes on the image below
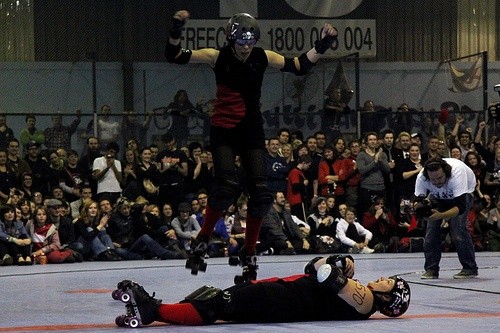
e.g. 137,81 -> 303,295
165,10 -> 338,272
0,86 -> 500,264
116,255 -> 411,328
414,158 -> 479,279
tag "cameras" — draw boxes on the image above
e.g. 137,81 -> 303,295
416,200 -> 433,220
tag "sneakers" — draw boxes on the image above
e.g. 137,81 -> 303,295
420,269 -> 438,279
359,246 -> 375,254
453,272 -> 478,278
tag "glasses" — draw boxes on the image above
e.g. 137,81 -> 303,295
236,39 -> 256,46
30,148 -> 38,150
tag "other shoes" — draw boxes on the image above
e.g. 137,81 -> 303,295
270,247 -> 278,254
3,254 -> 12,264
161,249 -> 178,259
100,251 -> 121,260
19,256 -> 30,264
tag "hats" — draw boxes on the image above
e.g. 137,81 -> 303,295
26,140 -> 38,149
46,199 -> 62,207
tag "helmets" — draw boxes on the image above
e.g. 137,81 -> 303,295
380,276 -> 411,318
226,12 -> 261,40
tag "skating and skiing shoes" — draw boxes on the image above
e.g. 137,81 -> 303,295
185,238 -> 209,275
229,246 -> 258,284
112,281 -> 161,328
